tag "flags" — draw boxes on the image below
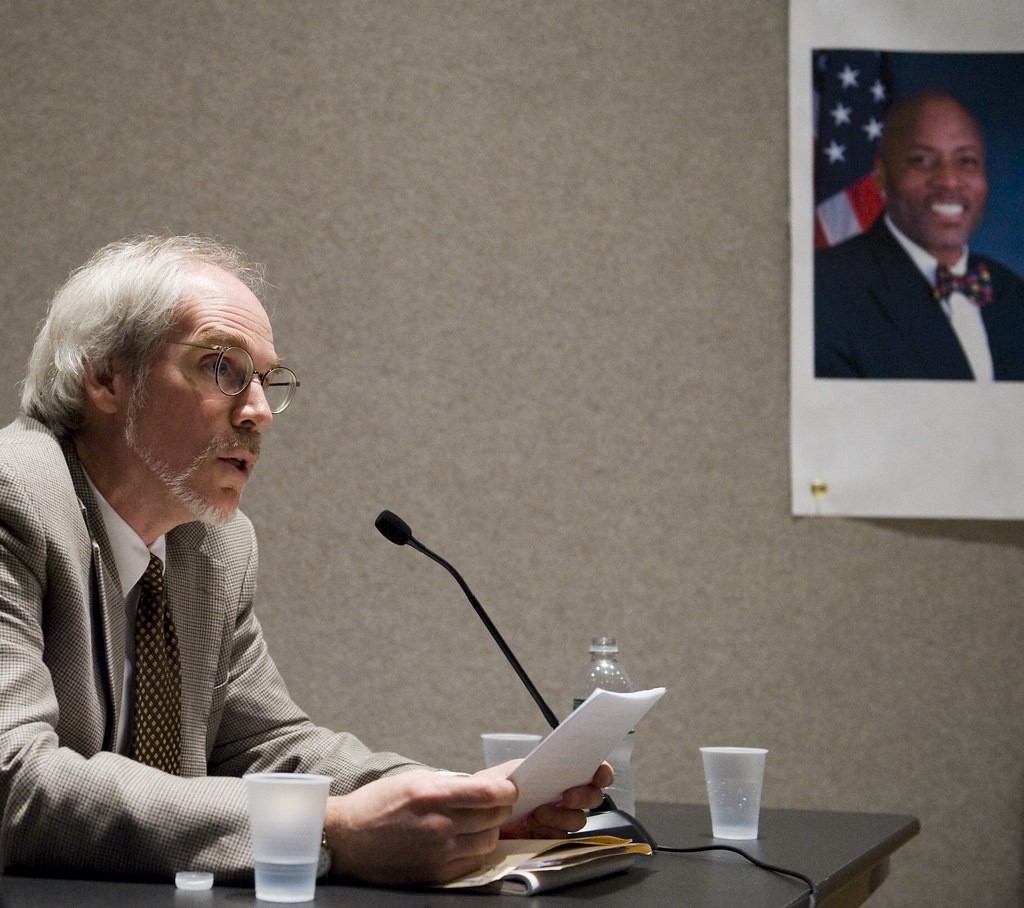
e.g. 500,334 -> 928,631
812,48 -> 888,246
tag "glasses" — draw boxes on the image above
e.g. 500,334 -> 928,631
152,334 -> 303,415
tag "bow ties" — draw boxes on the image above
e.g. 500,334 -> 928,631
931,261 -> 992,301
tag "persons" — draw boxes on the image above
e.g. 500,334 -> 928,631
814,82 -> 1023,383
0,235 -> 620,892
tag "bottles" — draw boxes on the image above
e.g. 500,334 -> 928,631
574,636 -> 636,818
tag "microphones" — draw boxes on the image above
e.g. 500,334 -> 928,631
373,509 -> 660,852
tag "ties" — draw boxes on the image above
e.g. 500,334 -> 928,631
133,554 -> 183,778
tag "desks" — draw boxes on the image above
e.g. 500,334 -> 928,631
0,799 -> 923,908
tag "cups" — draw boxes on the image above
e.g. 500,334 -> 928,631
479,733 -> 543,769
699,746 -> 769,840
242,772 -> 333,903
